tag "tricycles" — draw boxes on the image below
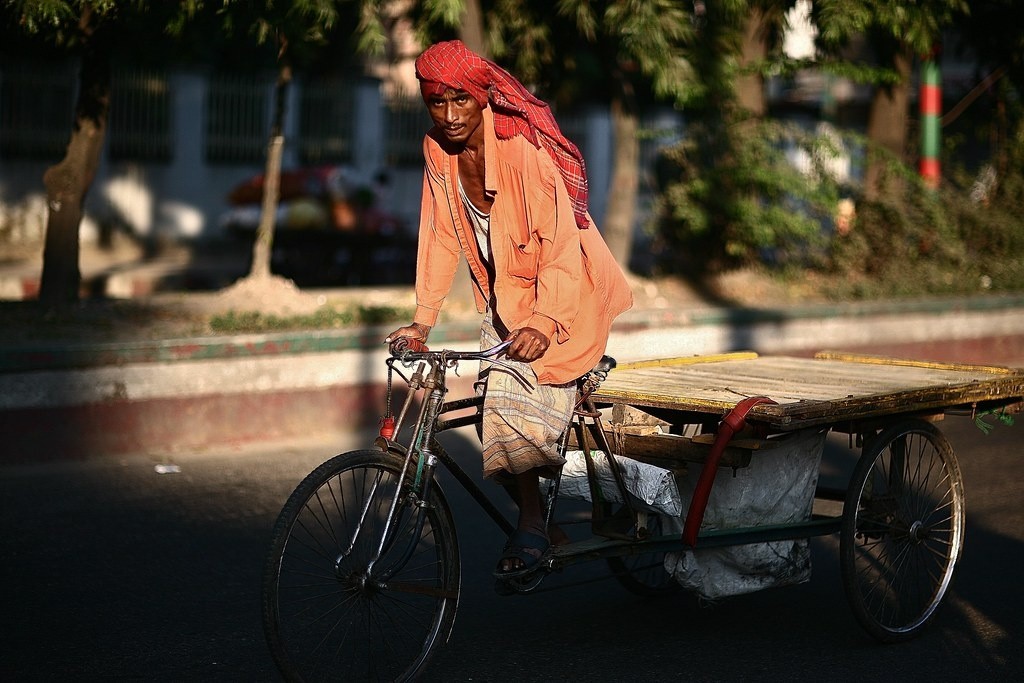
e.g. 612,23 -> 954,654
260,336 -> 1024,683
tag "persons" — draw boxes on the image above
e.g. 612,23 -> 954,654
383,39 -> 634,576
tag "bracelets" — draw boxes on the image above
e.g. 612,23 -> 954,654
413,325 -> 427,340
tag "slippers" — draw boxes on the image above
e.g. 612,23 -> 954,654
492,530 -> 551,579
495,579 -> 516,596
